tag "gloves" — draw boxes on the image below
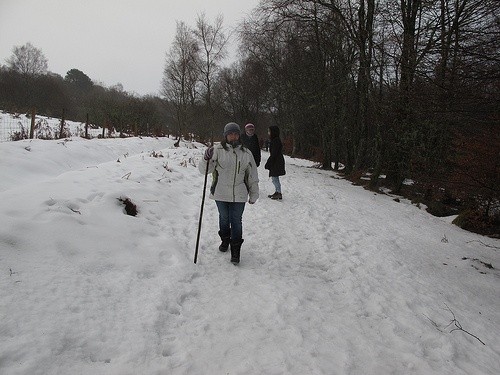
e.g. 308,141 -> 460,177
204,146 -> 214,160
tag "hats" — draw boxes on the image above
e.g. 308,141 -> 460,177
223,122 -> 240,136
245,123 -> 255,130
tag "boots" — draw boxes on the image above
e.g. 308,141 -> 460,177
230,239 -> 244,264
218,228 -> 231,252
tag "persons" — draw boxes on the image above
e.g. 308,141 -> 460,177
265,126 -> 286,200
264,140 -> 269,152
260,136 -> 263,149
239,123 -> 261,167
199,123 -> 260,265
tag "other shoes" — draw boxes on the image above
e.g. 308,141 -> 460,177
268,192 -> 282,200
